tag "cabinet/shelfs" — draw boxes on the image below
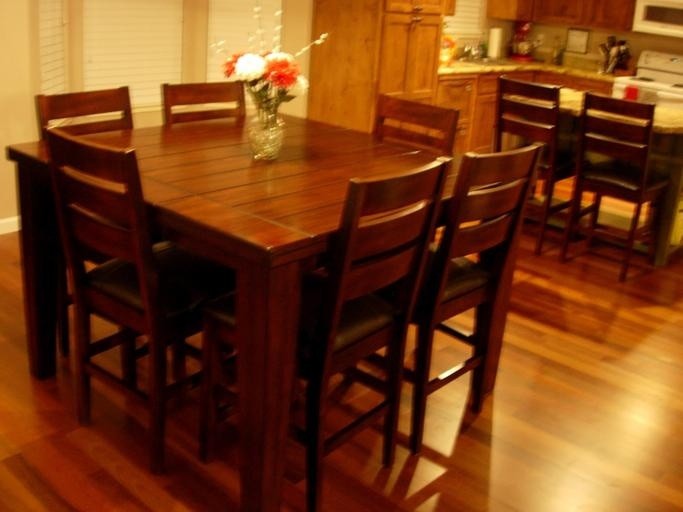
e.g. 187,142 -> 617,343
306,0 -> 636,201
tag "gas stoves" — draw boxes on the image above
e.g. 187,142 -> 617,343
614,76 -> 683,94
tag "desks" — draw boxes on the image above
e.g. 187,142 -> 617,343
503,79 -> 681,269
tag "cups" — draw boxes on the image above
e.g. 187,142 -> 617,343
623,86 -> 639,101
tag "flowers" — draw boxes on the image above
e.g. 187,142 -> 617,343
209,2 -> 329,130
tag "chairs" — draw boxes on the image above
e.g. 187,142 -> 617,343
36,83 -> 173,392
363,89 -> 465,156
308,137 -> 549,454
491,74 -> 587,264
556,91 -> 671,286
157,79 -> 245,125
197,151 -> 455,512
42,129 -> 245,477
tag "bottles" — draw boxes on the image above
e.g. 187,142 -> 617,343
598,35 -> 627,74
478,32 -> 487,58
